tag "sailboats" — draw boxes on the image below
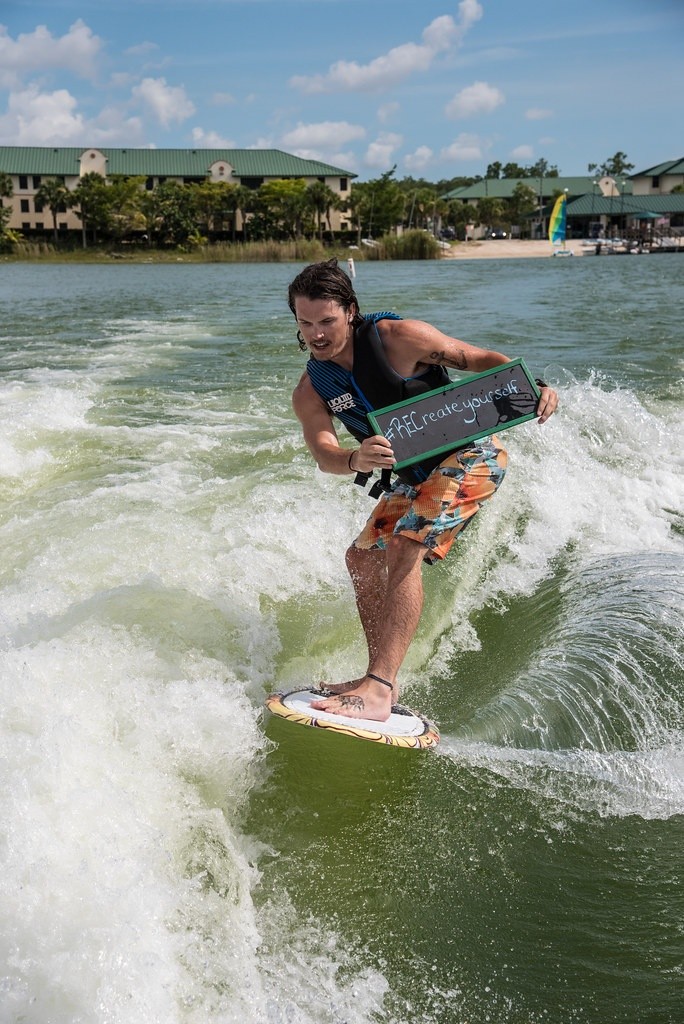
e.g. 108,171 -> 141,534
548,189 -> 574,257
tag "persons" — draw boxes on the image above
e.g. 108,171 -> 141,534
287,257 -> 560,722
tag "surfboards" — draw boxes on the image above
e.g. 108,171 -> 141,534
263,686 -> 441,750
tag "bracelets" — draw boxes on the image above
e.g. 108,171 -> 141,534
348,450 -> 359,472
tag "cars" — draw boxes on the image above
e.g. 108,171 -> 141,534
492,228 -> 506,240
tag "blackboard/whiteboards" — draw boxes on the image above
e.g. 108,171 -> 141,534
367,357 -> 543,471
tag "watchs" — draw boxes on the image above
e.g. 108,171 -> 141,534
535,379 -> 547,387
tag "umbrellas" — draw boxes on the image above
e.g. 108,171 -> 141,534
631,211 -> 663,219
